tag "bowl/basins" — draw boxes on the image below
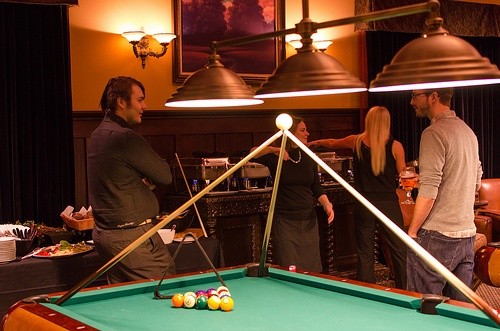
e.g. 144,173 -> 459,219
158,229 -> 175,244
14,239 -> 33,256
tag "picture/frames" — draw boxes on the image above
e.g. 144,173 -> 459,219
171,0 -> 285,85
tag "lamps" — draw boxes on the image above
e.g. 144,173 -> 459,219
164,0 -> 500,106
123,30 -> 177,69
287,39 -> 333,54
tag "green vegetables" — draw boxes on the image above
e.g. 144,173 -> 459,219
16,221 -> 76,250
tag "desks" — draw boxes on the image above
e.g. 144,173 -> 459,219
0,236 -> 218,324
1,261 -> 500,331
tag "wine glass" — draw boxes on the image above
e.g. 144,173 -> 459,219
399,166 -> 415,205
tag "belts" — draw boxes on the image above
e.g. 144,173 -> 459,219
94,217 -> 152,229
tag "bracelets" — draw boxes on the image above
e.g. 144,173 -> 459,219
323,202 -> 333,211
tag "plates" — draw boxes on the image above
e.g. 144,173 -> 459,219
174,237 -> 198,244
33,244 -> 93,258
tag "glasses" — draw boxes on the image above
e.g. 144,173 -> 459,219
411,92 -> 428,98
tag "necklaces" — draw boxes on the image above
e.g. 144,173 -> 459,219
284,146 -> 301,163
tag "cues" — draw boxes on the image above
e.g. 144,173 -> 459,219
52,130 -> 283,306
175,153 -> 208,237
284,129 -> 500,325
257,134 -> 287,277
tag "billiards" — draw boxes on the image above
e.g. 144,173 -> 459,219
196,286 -> 231,299
276,113 -> 293,130
184,292 -> 196,298
184,296 -> 195,308
220,297 -> 234,311
196,296 -> 207,308
172,294 -> 184,308
208,296 -> 221,310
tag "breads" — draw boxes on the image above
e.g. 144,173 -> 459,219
72,210 -> 94,220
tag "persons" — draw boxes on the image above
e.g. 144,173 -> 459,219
243,114 -> 335,274
86,75 -> 177,285
305,106 -> 406,290
398,87 -> 483,303
